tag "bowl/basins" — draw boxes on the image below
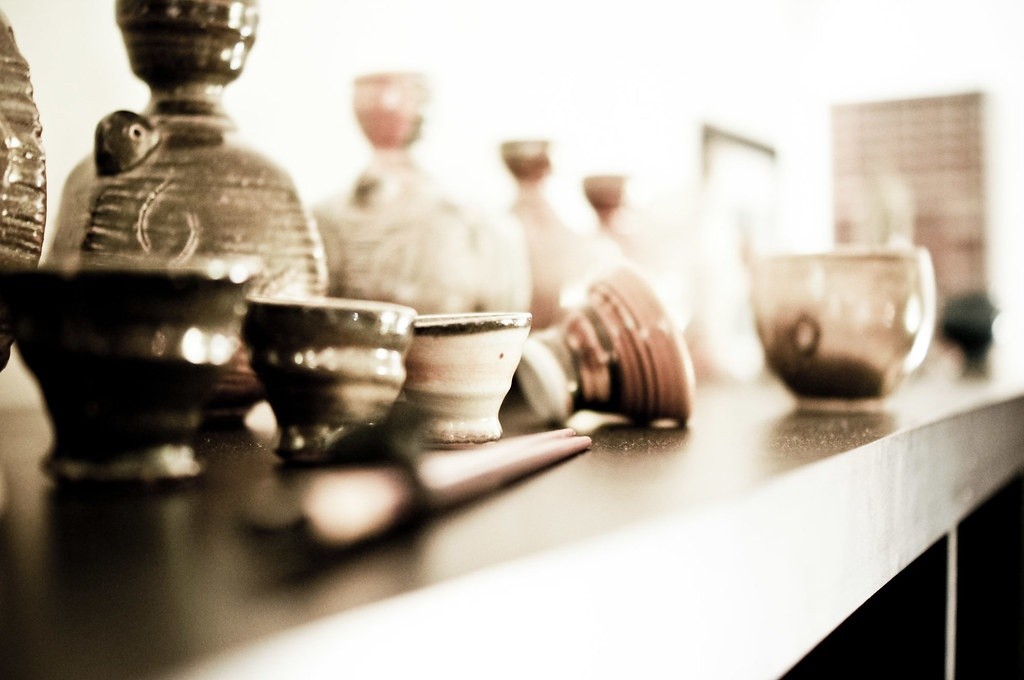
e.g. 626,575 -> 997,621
244,300 -> 415,457
0,259 -> 267,478
520,266 -> 693,428
758,247 -> 936,413
405,314 -> 532,443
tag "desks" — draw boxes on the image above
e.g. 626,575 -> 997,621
0,381 -> 1024,680
